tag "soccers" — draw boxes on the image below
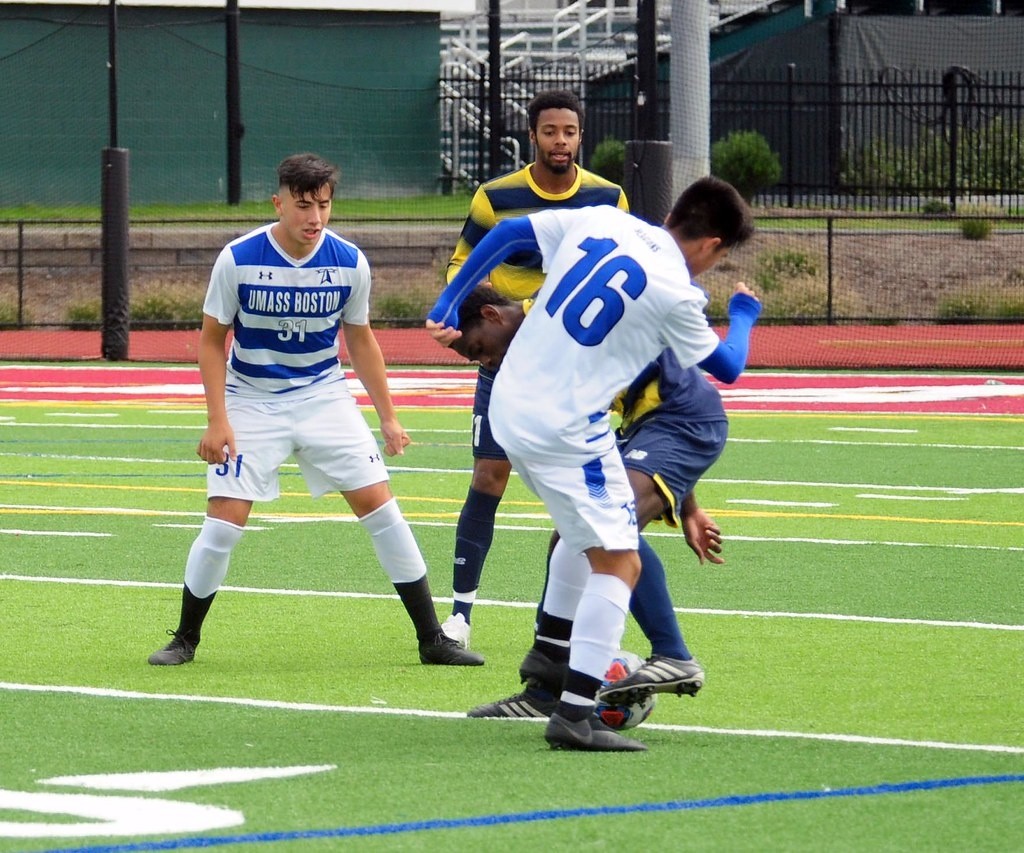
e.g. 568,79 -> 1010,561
593,648 -> 658,731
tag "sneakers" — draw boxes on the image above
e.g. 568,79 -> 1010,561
147,630 -> 198,666
467,693 -> 559,718
419,632 -> 485,667
442,612 -> 471,649
599,655 -> 705,708
545,712 -> 647,752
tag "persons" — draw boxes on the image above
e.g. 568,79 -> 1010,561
449,285 -> 729,718
425,176 -> 761,752
439,91 -> 631,651
148,154 -> 486,665
